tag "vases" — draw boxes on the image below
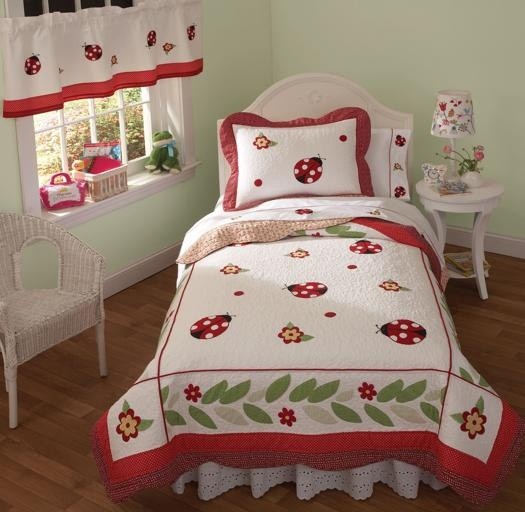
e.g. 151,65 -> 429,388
461,170 -> 485,189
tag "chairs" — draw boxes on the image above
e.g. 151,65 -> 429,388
0,210 -> 109,428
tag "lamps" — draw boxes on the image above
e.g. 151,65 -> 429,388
432,86 -> 474,185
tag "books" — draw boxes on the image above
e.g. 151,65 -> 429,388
445,257 -> 475,277
445,251 -> 491,272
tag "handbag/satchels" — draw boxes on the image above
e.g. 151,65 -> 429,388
40,172 -> 84,209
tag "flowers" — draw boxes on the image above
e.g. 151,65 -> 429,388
436,142 -> 485,173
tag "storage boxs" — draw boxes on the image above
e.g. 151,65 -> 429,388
72,161 -> 129,203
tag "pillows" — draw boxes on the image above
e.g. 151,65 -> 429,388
220,107 -> 374,211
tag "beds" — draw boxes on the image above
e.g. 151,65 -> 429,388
91,72 -> 521,503
